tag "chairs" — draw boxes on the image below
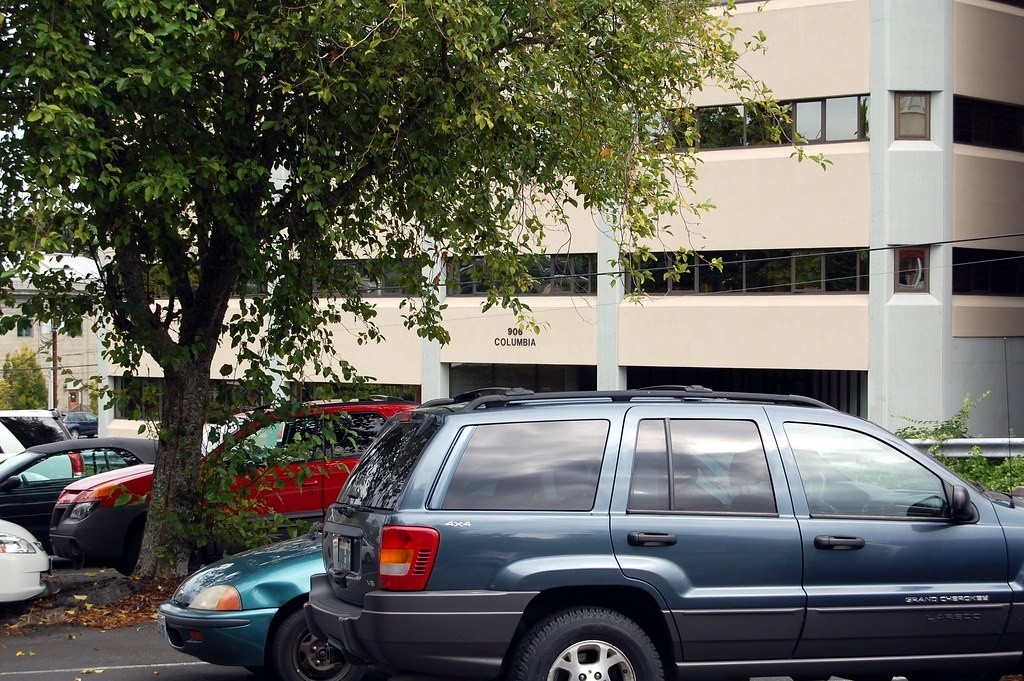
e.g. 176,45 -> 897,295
554,458 -> 584,501
676,449 -> 701,511
726,453 -> 763,511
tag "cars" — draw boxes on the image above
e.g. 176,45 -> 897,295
0,436 -> 160,567
59,411 -> 98,438
0,518 -> 51,606
155,452 -> 750,681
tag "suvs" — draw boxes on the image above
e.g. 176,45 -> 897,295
0,409 -> 87,483
299,384 -> 1024,681
45,392 -> 425,576
